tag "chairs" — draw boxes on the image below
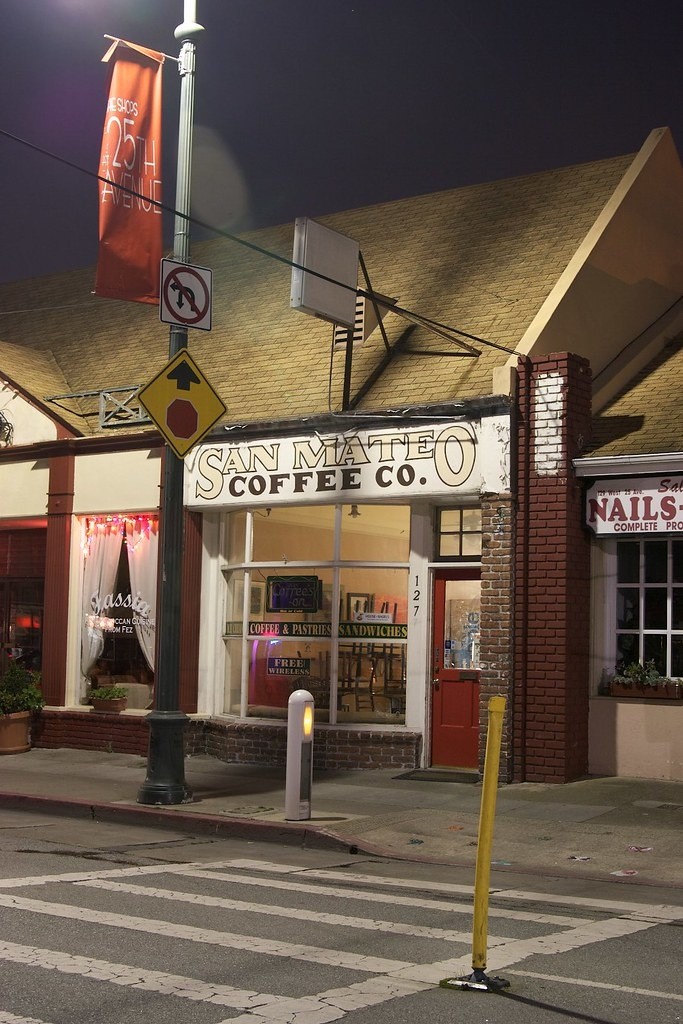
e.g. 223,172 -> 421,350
290,650 -> 381,712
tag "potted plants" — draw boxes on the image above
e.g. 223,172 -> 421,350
611,657 -> 683,700
87,687 -> 130,712
0,665 -> 47,756
598,667 -> 614,697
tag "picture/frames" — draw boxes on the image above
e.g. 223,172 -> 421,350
347,592 -> 370,622
231,579 -> 267,623
